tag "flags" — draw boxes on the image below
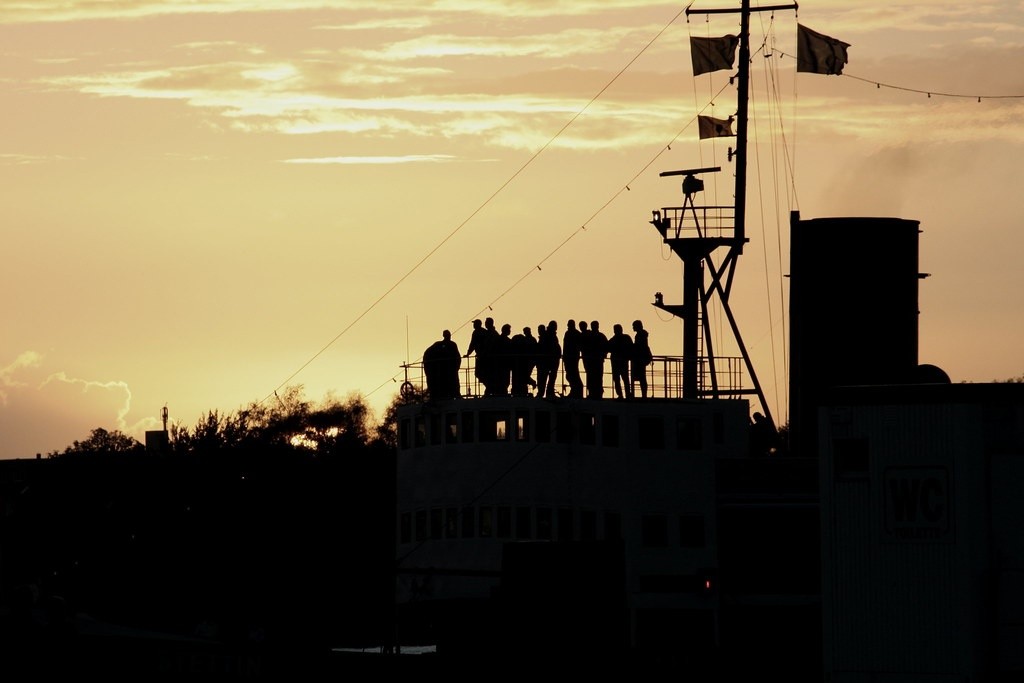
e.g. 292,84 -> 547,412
689,34 -> 739,76
697,116 -> 735,139
796,23 -> 851,76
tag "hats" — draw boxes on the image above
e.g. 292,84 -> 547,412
472,319 -> 482,326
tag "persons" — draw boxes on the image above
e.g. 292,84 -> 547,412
407,579 -> 418,604
423,319 -> 653,402
